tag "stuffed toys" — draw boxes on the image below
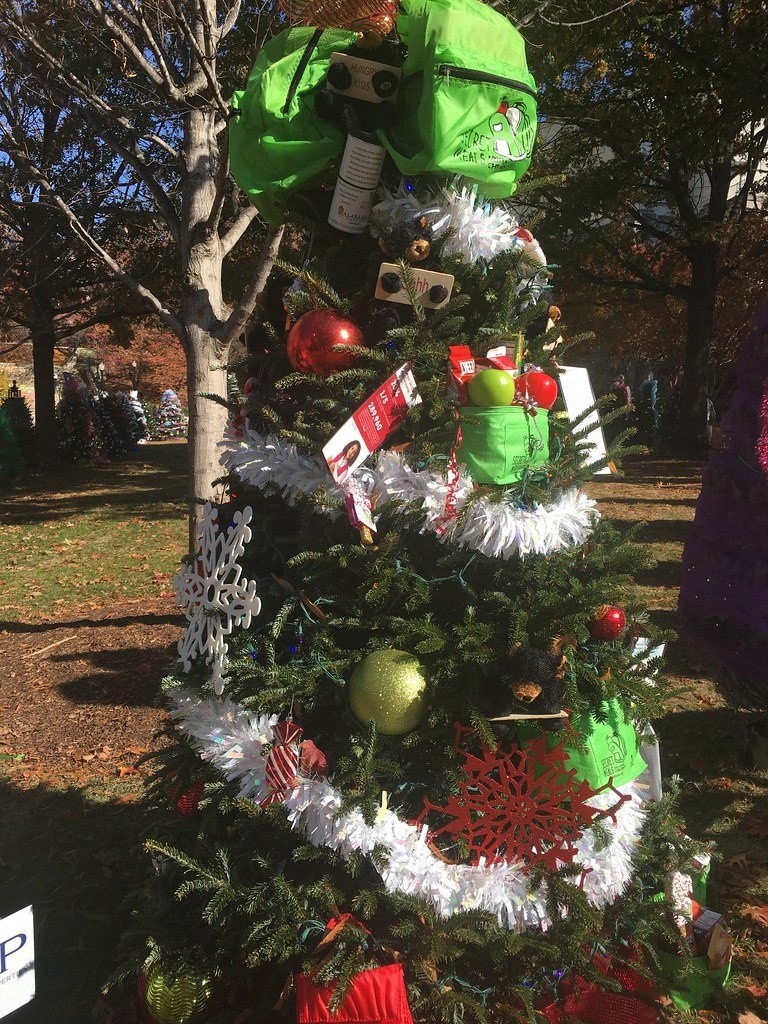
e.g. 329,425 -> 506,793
317,29 -> 407,126
374,217 -> 457,336
527,304 -> 565,361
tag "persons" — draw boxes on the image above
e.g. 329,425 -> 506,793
707,399 -> 716,442
612,375 -> 628,402
325,440 -> 361,483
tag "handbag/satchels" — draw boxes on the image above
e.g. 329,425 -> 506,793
295,913 -> 413,1024
453,405 -> 550,483
515,690 -> 649,803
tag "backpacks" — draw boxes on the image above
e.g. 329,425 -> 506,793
227,25 -> 361,229
375,0 -> 538,200
